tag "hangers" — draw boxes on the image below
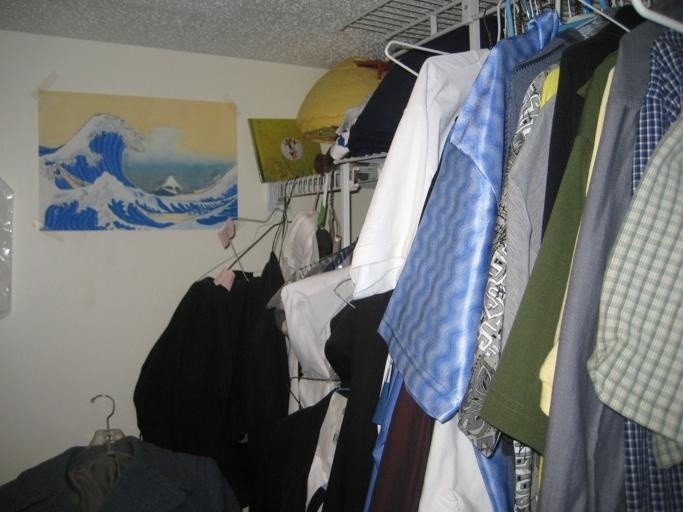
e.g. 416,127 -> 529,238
217,194 -> 291,301
61,393 -> 137,480
574,0 -> 623,44
384,40 -> 451,79
287,374 -> 340,410
333,279 -> 359,311
332,387 -> 355,404
480,0 -> 576,50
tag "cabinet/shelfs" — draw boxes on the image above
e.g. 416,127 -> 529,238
275,150 -> 386,283
340,0 -> 683,91
